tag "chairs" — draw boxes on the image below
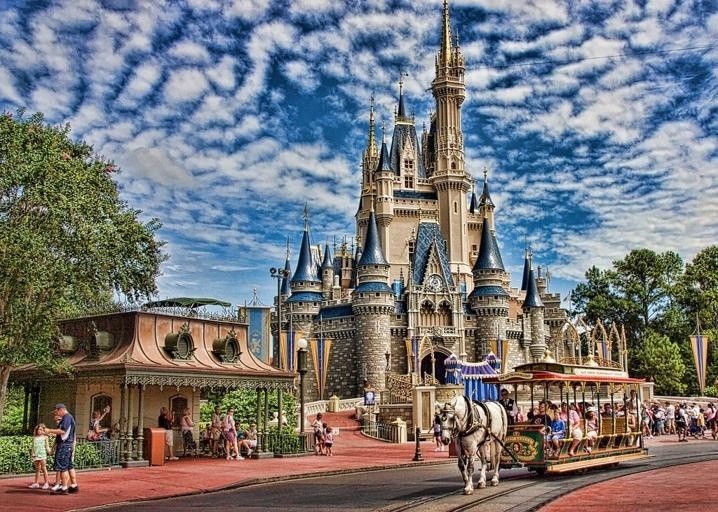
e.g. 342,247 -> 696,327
182,430 -> 196,455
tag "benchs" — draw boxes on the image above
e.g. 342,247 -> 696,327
545,416 -> 642,457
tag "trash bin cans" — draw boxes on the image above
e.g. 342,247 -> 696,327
142,428 -> 166,466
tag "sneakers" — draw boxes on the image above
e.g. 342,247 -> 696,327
51,484 -> 62,491
227,456 -> 233,460
49,488 -> 68,495
68,485 -> 79,493
235,456 -> 245,460
28,483 -> 40,489
169,456 -> 179,460
247,449 -> 253,456
42,483 -> 50,490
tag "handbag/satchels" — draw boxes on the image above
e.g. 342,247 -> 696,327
86,429 -> 103,441
220,422 -> 233,432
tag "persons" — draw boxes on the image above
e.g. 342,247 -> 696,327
499,388 -> 718,459
428,416 -> 444,452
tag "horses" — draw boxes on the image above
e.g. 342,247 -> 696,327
434,394 -> 508,494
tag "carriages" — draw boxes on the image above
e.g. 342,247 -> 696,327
433,360 -> 647,495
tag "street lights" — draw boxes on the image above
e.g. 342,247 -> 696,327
296,337 -> 309,453
410,351 -> 416,373
384,350 -> 391,372
269,266 -> 292,434
